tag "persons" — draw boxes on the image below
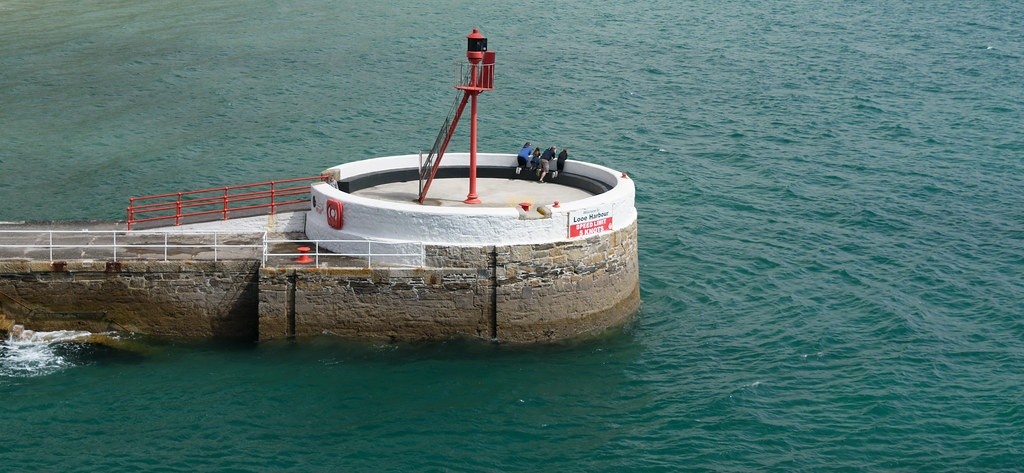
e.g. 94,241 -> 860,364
516,142 -> 532,174
529,146 -> 556,182
552,148 -> 567,178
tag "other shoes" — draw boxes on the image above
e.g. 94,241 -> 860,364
536,169 -> 540,176
516,167 -> 521,174
551,171 -> 558,178
540,179 -> 544,182
